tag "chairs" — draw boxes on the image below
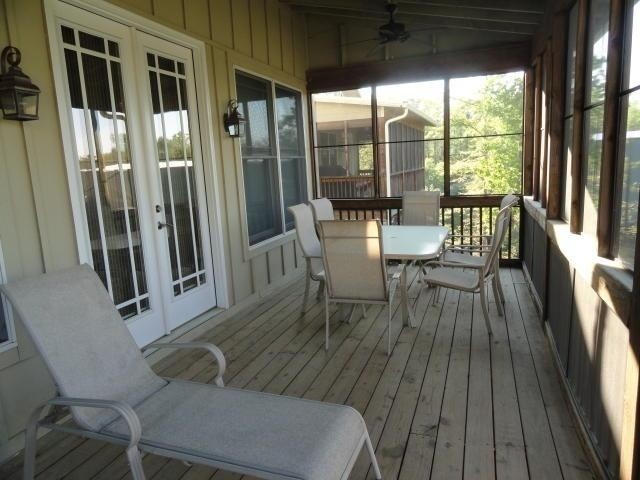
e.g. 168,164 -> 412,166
286,188 -> 523,356
0,262 -> 383,479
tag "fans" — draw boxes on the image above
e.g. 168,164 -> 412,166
336,3 -> 451,59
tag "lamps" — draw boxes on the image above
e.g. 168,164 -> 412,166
222,96 -> 247,139
1,41 -> 41,122
